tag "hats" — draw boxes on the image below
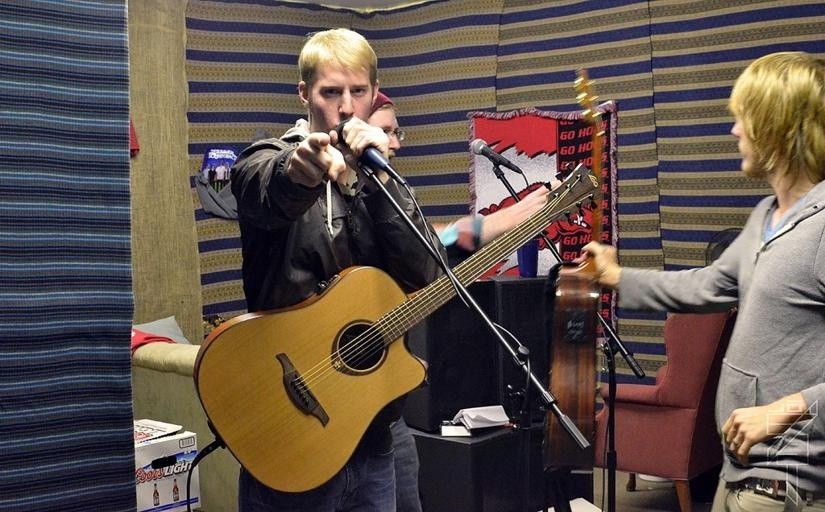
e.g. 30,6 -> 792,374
369,92 -> 394,116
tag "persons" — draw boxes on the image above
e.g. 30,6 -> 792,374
365,91 -> 564,512
571,51 -> 825,512
230,28 -> 448,512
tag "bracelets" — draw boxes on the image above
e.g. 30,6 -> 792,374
473,212 -> 483,252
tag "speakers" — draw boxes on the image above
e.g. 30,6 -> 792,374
489,274 -> 554,419
408,423 -> 548,512
405,277 -> 499,434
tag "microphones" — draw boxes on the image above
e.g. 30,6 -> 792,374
470,139 -> 523,174
335,118 -> 407,187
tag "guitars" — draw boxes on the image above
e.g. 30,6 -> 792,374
193,162 -> 601,493
540,67 -> 608,474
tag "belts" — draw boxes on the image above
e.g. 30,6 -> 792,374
739,477 -> 822,512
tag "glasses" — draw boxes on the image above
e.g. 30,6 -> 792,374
384,129 -> 406,141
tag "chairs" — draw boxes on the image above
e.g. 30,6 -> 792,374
592,307 -> 739,511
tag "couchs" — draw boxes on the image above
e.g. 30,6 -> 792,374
130,315 -> 240,512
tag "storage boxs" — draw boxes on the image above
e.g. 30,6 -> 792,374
132,418 -> 202,511
